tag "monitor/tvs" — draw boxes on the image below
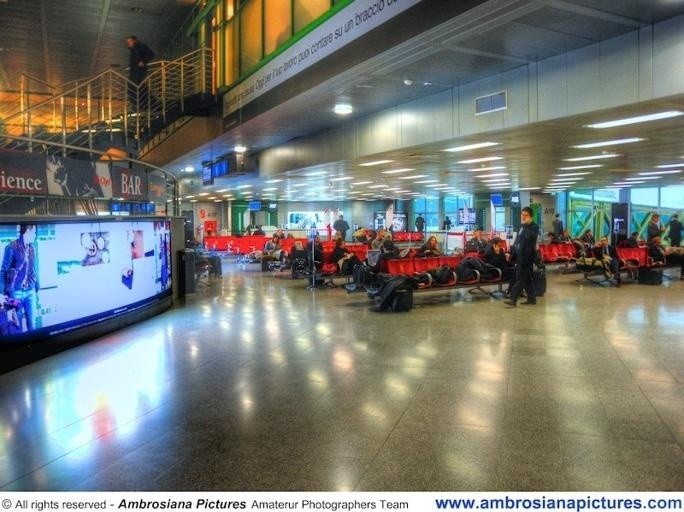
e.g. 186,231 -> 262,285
458,208 -> 477,224
490,194 -> 504,206
202,161 -> 214,185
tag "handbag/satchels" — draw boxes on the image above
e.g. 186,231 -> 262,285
413,271 -> 429,283
432,263 -> 453,284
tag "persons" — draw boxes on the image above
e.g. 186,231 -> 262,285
126,34 -> 161,113
246,207 -> 684,305
0,224 -> 37,334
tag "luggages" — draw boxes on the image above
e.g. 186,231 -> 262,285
390,277 -> 413,310
353,264 -> 372,289
533,270 -> 546,296
638,266 -> 663,285
292,258 -> 306,279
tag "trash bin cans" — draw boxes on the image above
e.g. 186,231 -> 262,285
178,248 -> 196,302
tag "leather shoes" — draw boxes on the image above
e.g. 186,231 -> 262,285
520,300 -> 536,304
504,300 -> 516,305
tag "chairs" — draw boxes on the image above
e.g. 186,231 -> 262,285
203,231 -> 664,294
